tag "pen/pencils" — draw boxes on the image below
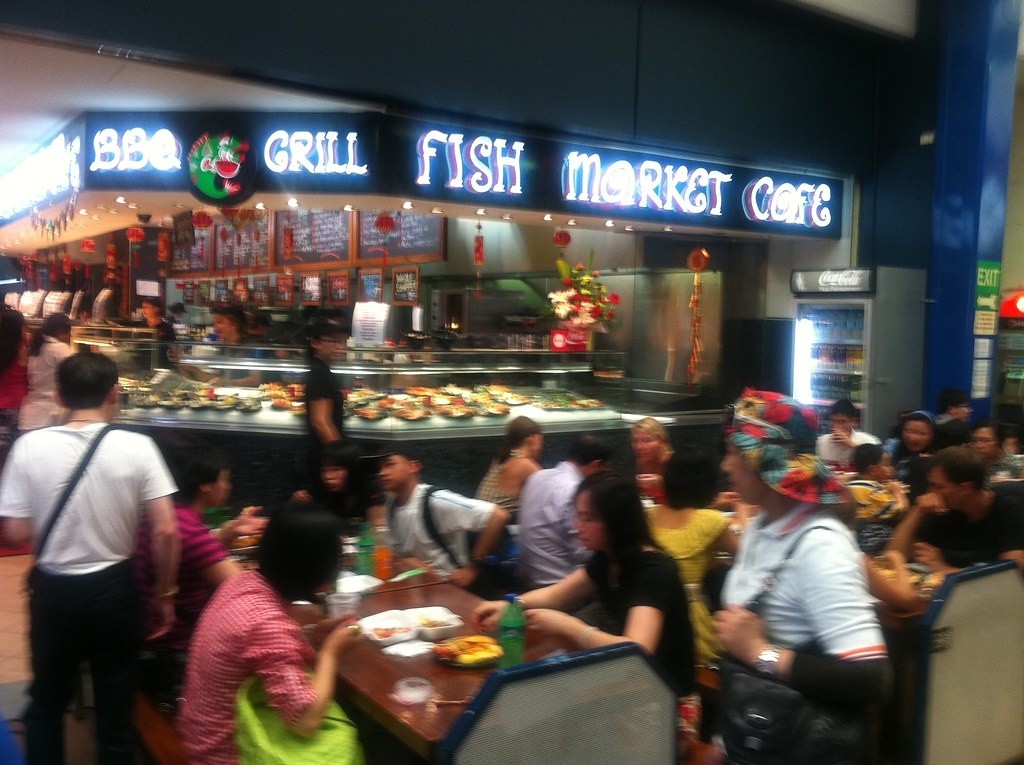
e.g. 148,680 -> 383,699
432,699 -> 470,705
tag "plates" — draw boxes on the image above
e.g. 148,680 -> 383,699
434,654 -> 502,671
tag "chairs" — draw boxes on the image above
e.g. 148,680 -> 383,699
908,560 -> 1024,765
430,640 -> 679,765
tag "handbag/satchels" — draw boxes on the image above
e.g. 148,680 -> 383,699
235,671 -> 364,765
715,659 -> 812,765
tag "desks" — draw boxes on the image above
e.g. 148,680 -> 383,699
289,544 -> 583,759
716,536 -> 938,631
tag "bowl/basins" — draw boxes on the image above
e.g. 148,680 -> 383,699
357,609 -> 422,647
404,605 -> 464,642
337,575 -> 385,596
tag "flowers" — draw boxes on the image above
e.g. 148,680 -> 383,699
548,249 -> 621,335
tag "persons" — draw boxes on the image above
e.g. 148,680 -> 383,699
713,386 -> 893,765
0,353 -> 181,765
361,414 -> 750,668
0,303 -> 76,488
471,472 -> 700,699
175,501 -> 364,765
301,323 -> 355,500
165,296 -> 347,386
103,297 -> 193,371
817,388 -> 1024,764
132,439 -> 512,653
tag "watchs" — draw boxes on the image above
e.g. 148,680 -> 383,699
757,644 -> 779,675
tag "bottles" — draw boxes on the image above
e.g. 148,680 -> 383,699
372,518 -> 395,580
496,593 -> 525,671
357,520 -> 376,577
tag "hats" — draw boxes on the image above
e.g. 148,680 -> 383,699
306,308 -> 352,334
358,441 -> 423,460
725,386 -> 854,503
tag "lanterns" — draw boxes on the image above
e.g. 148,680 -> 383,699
19,208 -> 394,287
552,228 -> 573,251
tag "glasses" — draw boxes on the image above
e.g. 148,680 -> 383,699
725,405 -> 788,439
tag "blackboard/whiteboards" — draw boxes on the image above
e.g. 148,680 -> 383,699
196,280 -> 211,308
357,269 -> 383,304
231,277 -> 250,306
181,278 -> 200,308
299,273 -> 323,305
252,275 -> 271,307
326,272 -> 350,306
214,279 -> 230,308
274,273 -> 294,307
391,267 -> 419,306
162,209 -> 447,278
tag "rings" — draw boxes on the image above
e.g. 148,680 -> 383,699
347,624 -> 360,635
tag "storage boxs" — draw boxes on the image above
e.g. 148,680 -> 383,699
550,331 -> 591,352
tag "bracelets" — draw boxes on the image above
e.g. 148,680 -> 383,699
156,587 -> 182,598
507,593 -> 525,608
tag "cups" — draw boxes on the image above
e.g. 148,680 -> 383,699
327,592 -> 362,618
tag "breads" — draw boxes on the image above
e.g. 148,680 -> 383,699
433,635 -> 504,664
